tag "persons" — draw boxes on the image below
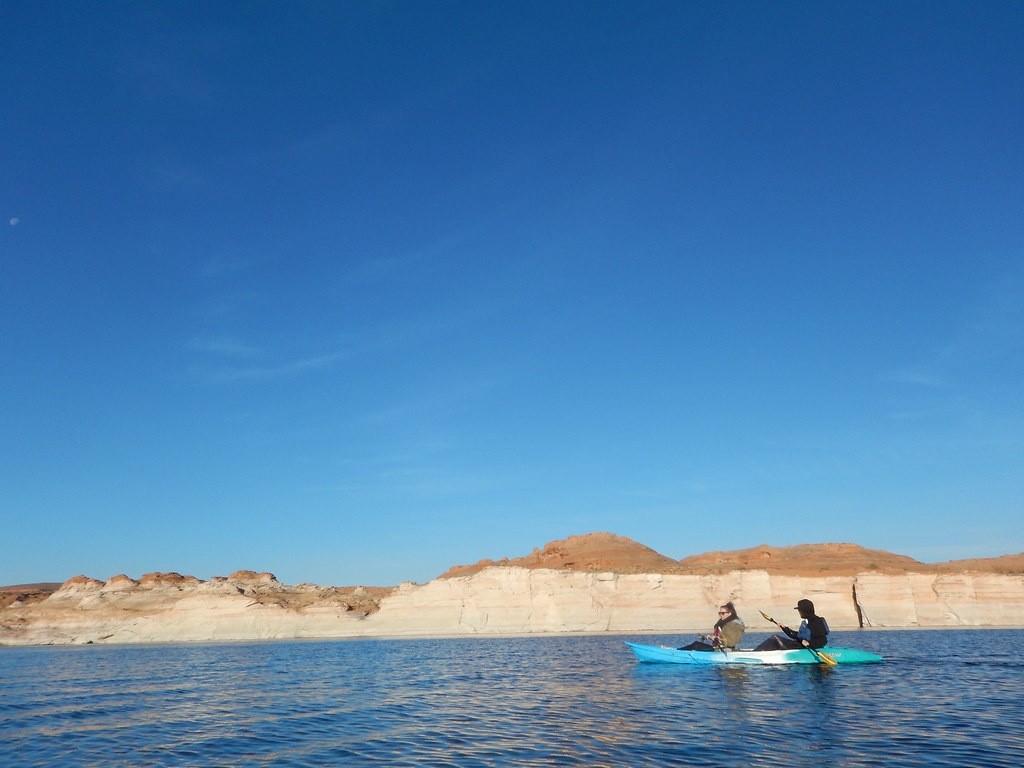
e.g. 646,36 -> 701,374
660,602 -> 745,652
753,599 -> 828,651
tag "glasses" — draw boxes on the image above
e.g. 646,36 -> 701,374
718,612 -> 730,615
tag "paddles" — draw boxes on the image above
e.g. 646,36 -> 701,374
758,609 -> 838,668
696,632 -> 729,657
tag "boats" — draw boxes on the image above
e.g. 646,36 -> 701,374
624,641 -> 883,664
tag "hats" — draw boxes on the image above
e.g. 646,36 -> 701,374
794,599 -> 814,611
